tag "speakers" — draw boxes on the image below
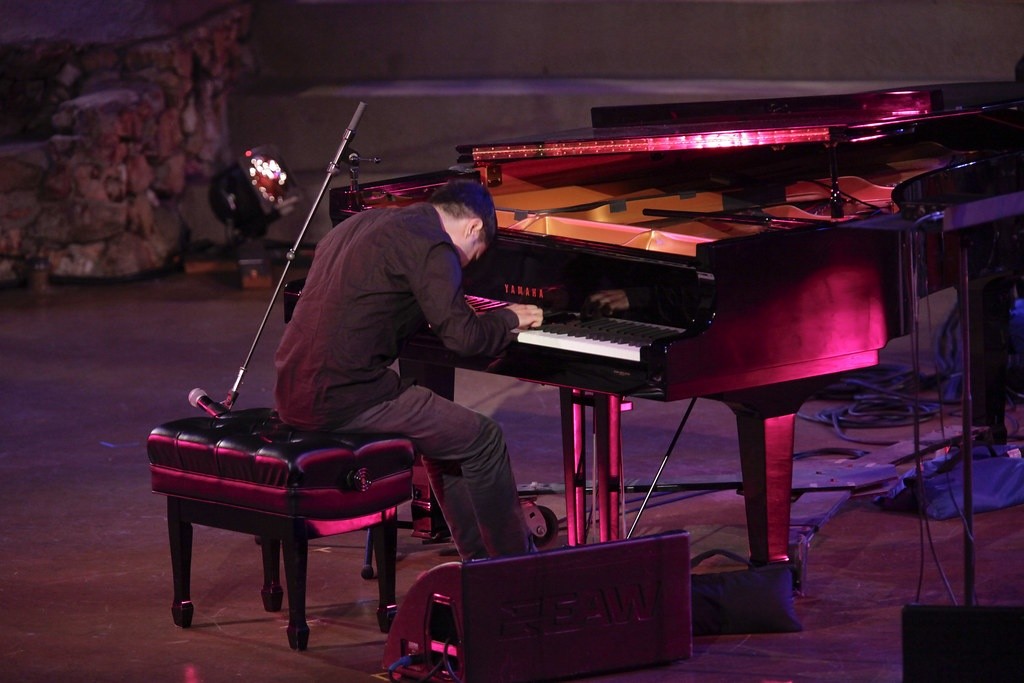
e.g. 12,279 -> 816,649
378,528 -> 693,683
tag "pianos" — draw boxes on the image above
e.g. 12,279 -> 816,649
280,81 -> 945,604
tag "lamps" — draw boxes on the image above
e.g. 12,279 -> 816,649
206,142 -> 302,257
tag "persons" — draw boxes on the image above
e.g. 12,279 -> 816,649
592,282 -> 655,313
272,179 -> 545,564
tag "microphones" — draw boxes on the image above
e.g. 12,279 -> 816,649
187,388 -> 225,418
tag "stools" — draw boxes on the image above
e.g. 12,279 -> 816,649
146,408 -> 418,653
